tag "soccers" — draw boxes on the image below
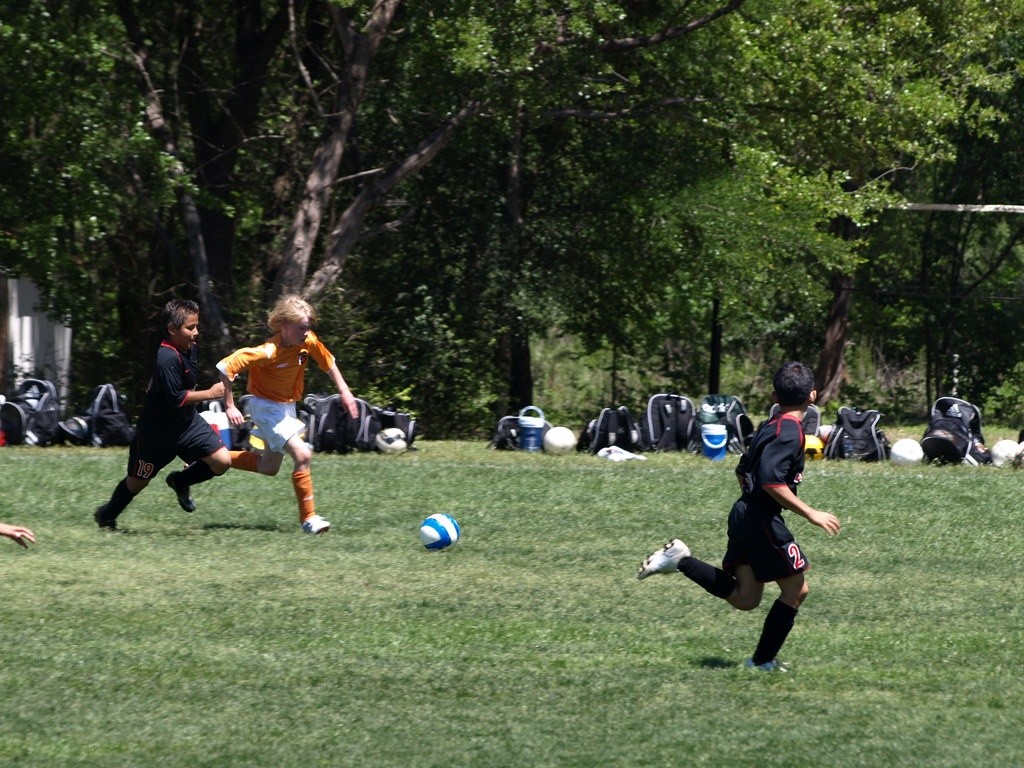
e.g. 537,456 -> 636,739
890,438 -> 923,467
375,428 -> 407,455
804,435 -> 824,462
419,513 -> 460,552
991,439 -> 1021,467
542,426 -> 578,454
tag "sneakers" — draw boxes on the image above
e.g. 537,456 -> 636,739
636,539 -> 692,580
303,515 -> 331,534
166,471 -> 197,512
93,507 -> 117,529
746,657 -> 786,671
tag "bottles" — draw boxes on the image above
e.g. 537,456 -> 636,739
843,435 -> 852,458
973,437 -> 986,453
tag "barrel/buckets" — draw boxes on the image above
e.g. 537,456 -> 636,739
700,424 -> 728,460
199,401 -> 232,450
518,406 -> 544,451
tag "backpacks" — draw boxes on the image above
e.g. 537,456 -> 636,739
494,386 -> 997,471
0,377 -> 422,452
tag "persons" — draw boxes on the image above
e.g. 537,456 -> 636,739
94,300 -> 232,531
0,523 -> 35,549
638,361 -> 840,666
216,297 -> 359,533
